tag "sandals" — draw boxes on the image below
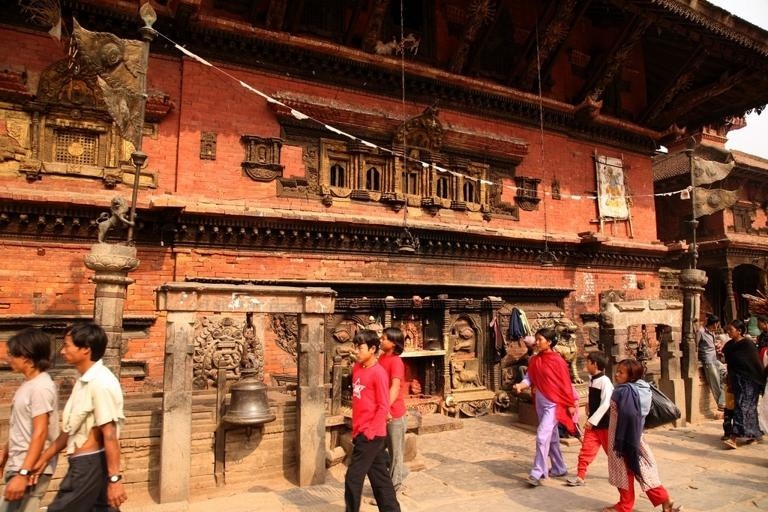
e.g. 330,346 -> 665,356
661,499 -> 679,512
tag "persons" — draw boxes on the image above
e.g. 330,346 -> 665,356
601,360 -> 682,512
699,317 -> 727,411
371,328 -> 407,505
569,351 -> 614,485
511,329 -> 577,485
755,316 -> 768,354
0,332 -> 58,512
345,330 -> 402,512
32,324 -> 127,512
722,320 -> 764,448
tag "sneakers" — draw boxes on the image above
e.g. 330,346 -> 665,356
743,437 -> 767,448
567,474 -> 587,488
723,437 -> 737,453
525,474 -> 538,487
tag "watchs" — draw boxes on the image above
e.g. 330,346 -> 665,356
108,474 -> 125,486
18,468 -> 31,478
